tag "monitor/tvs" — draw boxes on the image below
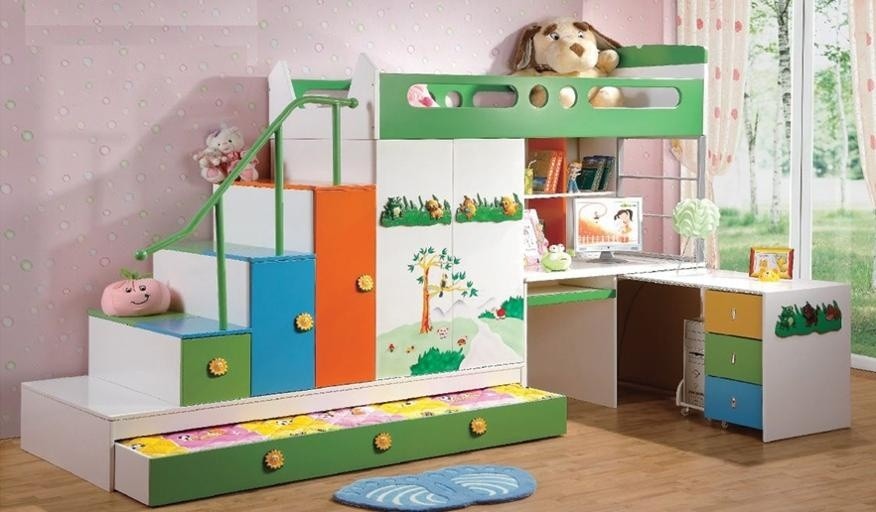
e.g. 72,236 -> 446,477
573,194 -> 645,265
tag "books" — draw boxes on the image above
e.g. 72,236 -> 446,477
530,150 -> 564,193
579,155 -> 615,191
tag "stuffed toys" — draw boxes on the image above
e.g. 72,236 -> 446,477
100,267 -> 172,315
193,125 -> 258,184
542,245 -> 572,271
510,17 -> 623,108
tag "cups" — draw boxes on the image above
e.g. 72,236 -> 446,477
524,169 -> 534,195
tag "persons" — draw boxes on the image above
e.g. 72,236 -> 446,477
566,162 -> 582,193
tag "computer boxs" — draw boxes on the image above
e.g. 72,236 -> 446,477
682,317 -> 707,410
